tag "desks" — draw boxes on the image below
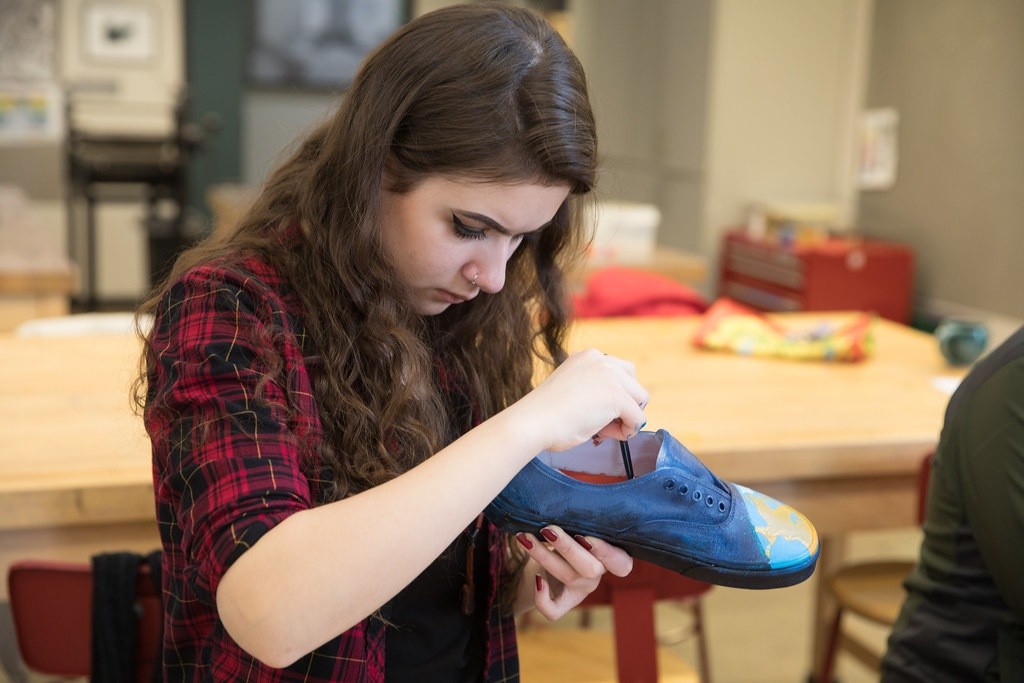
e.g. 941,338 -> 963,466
0,313 -> 963,683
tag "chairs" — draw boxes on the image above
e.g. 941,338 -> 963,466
8,560 -> 162,683
815,454 -> 934,683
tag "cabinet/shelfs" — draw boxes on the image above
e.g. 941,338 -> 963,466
720,224 -> 914,326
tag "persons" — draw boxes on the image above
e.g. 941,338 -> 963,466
133,1 -> 647,683
877,323 -> 1024,683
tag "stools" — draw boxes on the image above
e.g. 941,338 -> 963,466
579,555 -> 716,683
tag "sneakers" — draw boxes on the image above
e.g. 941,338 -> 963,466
485,428 -> 821,589
